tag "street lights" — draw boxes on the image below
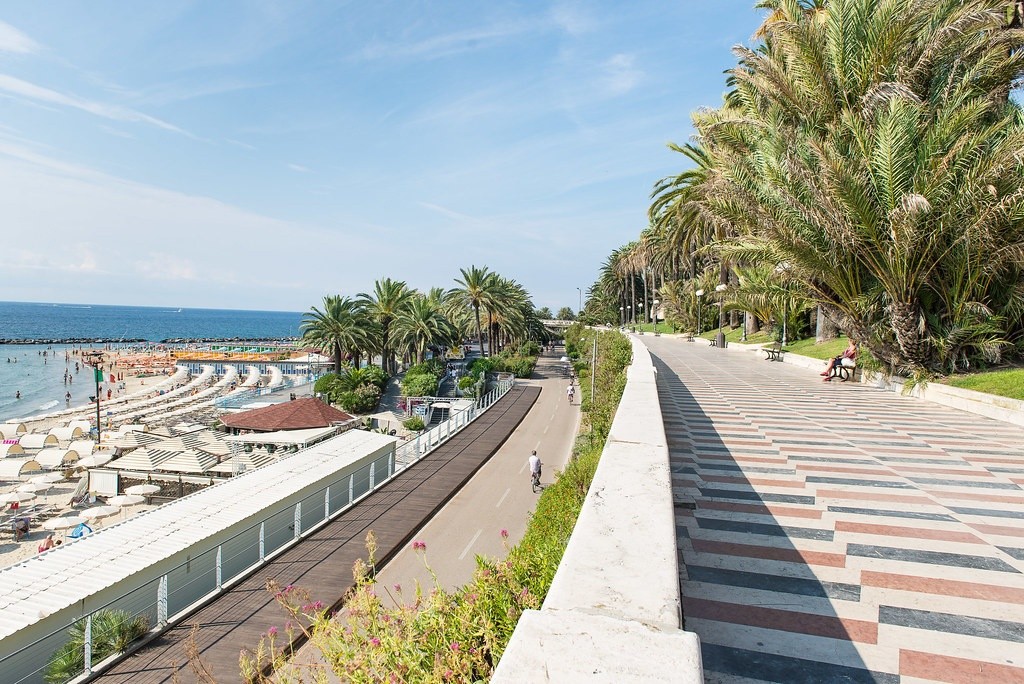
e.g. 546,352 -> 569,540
627,306 -> 631,328
696,289 -> 704,335
671,297 -> 677,332
619,307 -> 625,328
638,302 -> 643,330
653,300 -> 659,330
739,275 -> 747,342
776,260 -> 794,347
715,284 -> 727,332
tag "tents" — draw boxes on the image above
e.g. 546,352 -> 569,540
84,350 -> 104,357
0,424 -> 293,505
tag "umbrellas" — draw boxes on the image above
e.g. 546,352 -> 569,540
124,483 -> 161,513
5,364 -> 307,424
76,454 -> 112,467
0,491 -> 37,516
81,505 -> 121,528
32,473 -> 64,500
109,495 -> 145,519
43,516 -> 89,542
16,483 -> 54,507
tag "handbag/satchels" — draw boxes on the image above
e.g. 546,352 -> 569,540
841,357 -> 855,368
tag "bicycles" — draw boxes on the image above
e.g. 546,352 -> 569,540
529,474 -> 541,493
568,396 -> 574,405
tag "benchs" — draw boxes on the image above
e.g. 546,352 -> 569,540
709,334 -> 718,347
761,341 -> 783,361
825,362 -> 856,382
686,332 -> 694,342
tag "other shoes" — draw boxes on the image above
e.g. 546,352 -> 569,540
820,372 -> 829,376
823,377 -> 831,381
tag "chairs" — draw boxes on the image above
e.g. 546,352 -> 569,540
0,501 -> 59,538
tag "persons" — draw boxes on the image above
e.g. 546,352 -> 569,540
44,359 -> 47,364
65,391 -> 71,403
63,373 -> 67,385
7,345 -> 207,363
37,534 -> 58,552
100,365 -> 104,373
550,343 -> 554,352
68,374 -> 72,385
15,391 -> 20,398
65,368 -> 69,374
15,519 -> 28,542
570,372 -> 574,384
566,383 -> 575,402
820,338 -> 856,382
109,364 -> 112,371
530,450 -> 542,485
107,389 -> 112,399
76,367 -> 79,374
13,358 -> 17,363
